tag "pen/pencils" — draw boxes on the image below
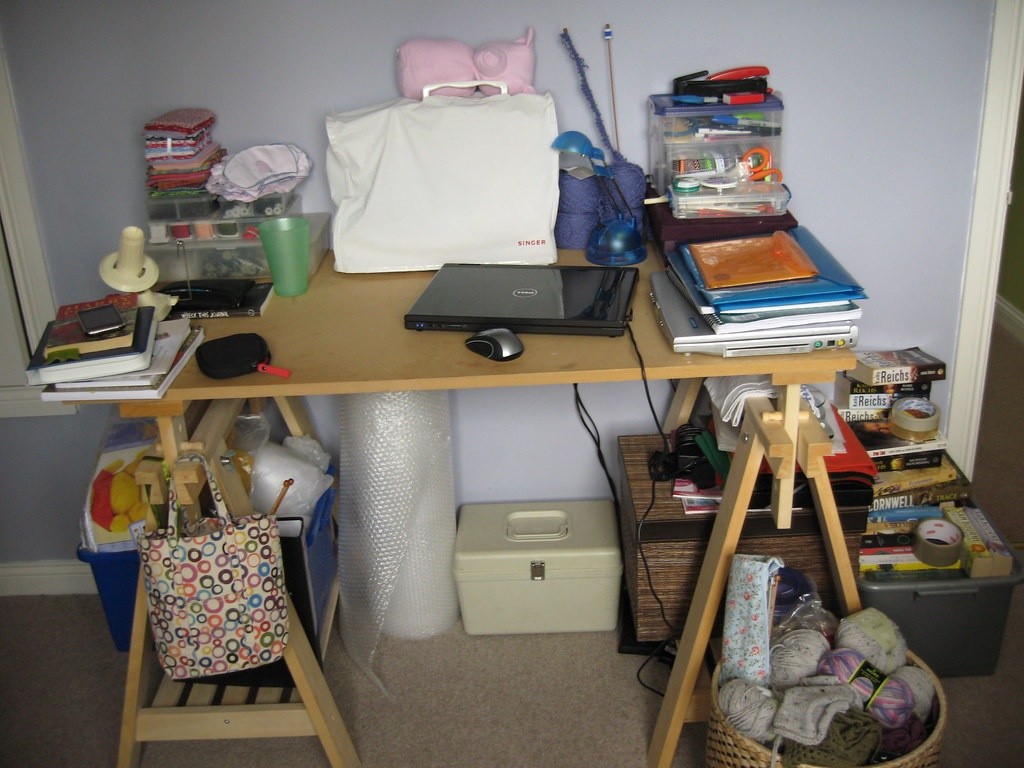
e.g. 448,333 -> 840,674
694,115 -> 781,135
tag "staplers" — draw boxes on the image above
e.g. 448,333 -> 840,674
708,66 -> 773,94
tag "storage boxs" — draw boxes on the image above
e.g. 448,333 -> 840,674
141,190 -> 332,286
613,433 -> 871,648
75,461 -> 338,654
852,496 -> 1024,682
452,499 -> 622,636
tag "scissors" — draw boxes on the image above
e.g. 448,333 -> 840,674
742,147 -> 782,192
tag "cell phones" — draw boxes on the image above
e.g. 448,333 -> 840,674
75,302 -> 127,336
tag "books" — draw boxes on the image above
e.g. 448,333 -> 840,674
830,347 -> 975,573
154,278 -> 275,321
24,292 -> 206,403
667,429 -> 802,515
665,226 -> 868,335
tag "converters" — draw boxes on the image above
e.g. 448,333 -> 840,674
676,427 -> 707,469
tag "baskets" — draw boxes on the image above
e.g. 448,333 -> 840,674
703,622 -> 948,768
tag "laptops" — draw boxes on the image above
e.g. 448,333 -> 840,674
405,263 -> 640,338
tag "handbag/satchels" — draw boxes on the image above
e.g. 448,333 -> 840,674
136,450 -> 289,681
322,90 -> 561,275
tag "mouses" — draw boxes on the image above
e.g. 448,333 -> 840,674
465,328 -> 524,362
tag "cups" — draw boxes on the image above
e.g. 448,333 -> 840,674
258,217 -> 311,296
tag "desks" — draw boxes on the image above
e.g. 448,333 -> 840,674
41,241 -> 895,768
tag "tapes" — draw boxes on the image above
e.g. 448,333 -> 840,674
911,519 -> 964,567
887,396 -> 940,442
